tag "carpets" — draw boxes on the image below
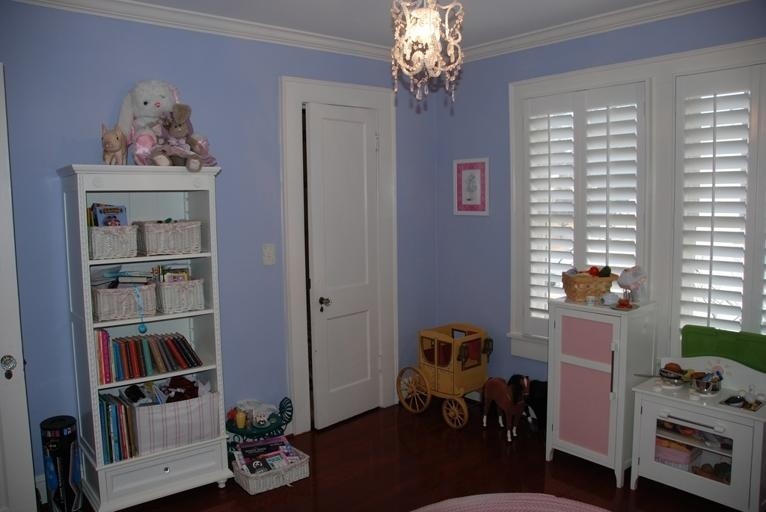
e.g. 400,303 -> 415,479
414,492 -> 610,512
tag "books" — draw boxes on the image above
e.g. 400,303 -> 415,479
99,392 -> 133,467
92,260 -> 192,289
93,329 -> 202,387
87,203 -> 128,228
235,436 -> 301,476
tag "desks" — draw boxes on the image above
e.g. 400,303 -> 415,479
225,412 -> 287,442
631,357 -> 766,512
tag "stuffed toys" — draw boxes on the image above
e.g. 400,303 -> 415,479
99,79 -> 218,171
165,377 -> 199,404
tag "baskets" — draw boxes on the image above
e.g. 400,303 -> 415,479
231,443 -> 310,496
91,281 -> 159,323
133,219 -> 202,257
89,223 -> 139,261
562,268 -> 620,304
154,278 -> 206,313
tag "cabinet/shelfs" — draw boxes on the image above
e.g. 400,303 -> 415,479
546,299 -> 656,488
55,164 -> 235,512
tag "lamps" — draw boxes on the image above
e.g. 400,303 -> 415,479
389,0 -> 464,102
